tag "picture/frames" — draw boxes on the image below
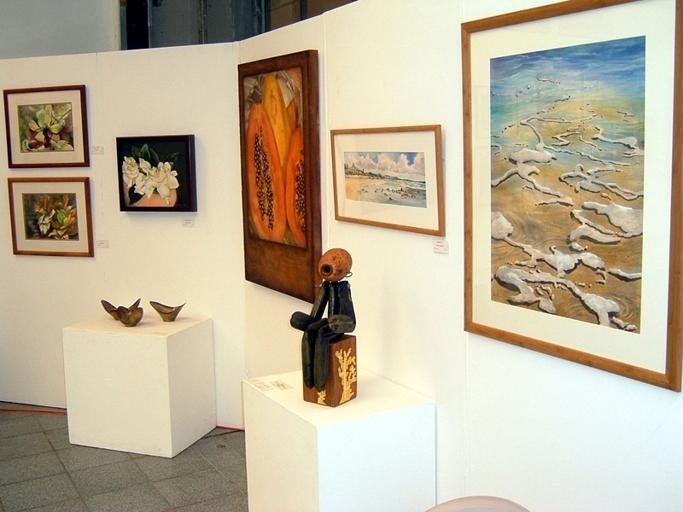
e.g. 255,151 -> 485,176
461,15 -> 683,394
330,124 -> 446,238
6,177 -> 95,258
1,85 -> 88,169
113,135 -> 199,215
238,49 -> 321,296
